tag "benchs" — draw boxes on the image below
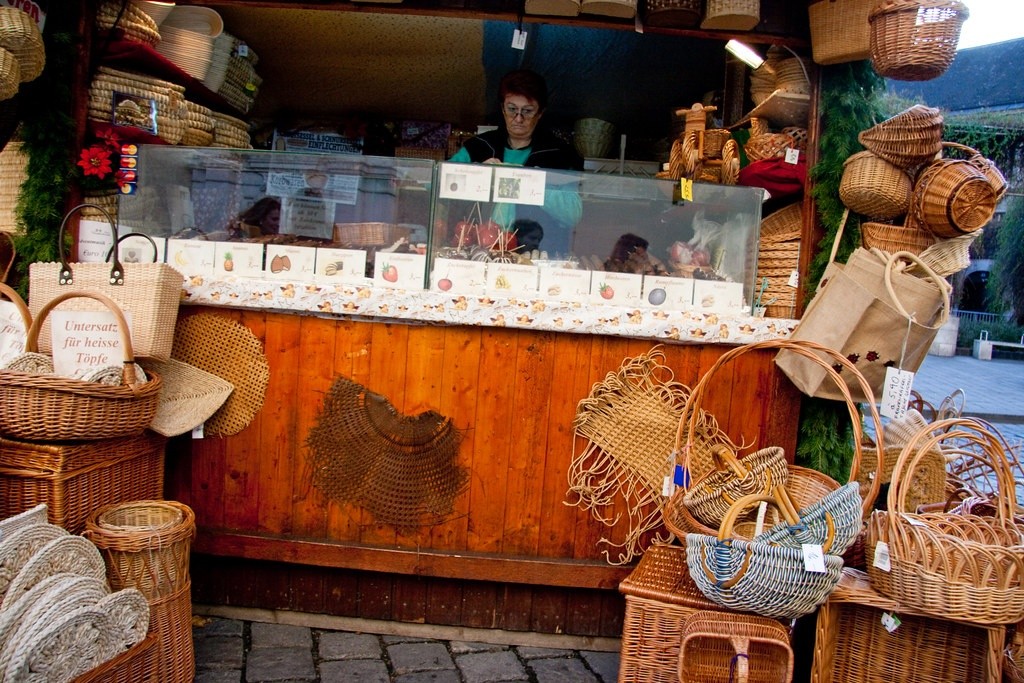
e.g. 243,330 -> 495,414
972,330 -> 1024,359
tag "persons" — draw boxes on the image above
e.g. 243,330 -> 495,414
603,233 -> 650,274
446,72 -> 582,258
230,196 -> 281,243
511,218 -> 543,253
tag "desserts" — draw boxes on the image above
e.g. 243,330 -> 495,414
439,200 -> 527,262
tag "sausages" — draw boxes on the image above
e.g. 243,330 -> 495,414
579,245 -> 668,276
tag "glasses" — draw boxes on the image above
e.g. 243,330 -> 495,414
501,102 -> 539,122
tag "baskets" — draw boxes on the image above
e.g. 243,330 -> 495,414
0,0 -> 1024,683
